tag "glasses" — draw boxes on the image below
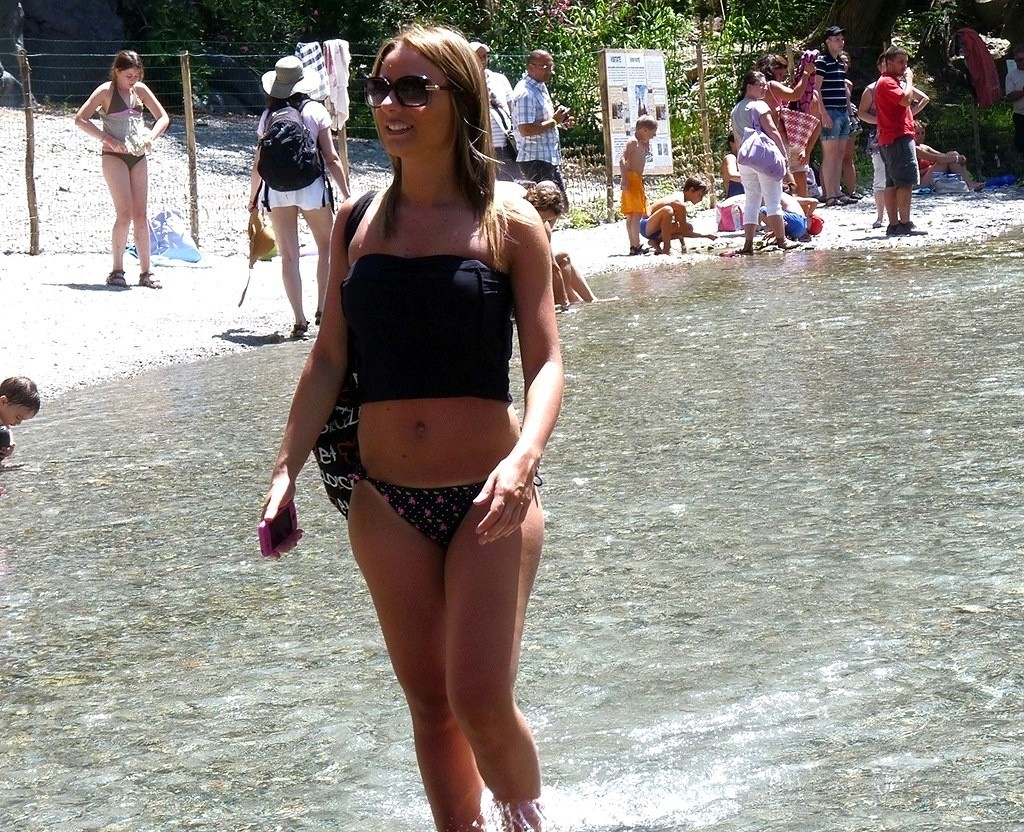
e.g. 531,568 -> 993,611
363,75 -> 465,107
773,65 -> 787,69
1016,58 -> 1024,62
752,83 -> 768,90
531,62 -> 554,70
915,131 -> 924,135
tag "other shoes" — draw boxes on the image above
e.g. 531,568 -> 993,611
844,192 -> 863,199
886,221 -> 928,236
292,321 -> 310,340
716,193 -> 745,232
314,306 -> 322,325
872,219 -> 882,228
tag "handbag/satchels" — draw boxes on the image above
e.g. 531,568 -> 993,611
737,100 -> 787,181
780,107 -> 820,152
506,131 -> 518,161
310,189 -> 368,522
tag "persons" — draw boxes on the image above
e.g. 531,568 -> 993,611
259,28 -> 573,832
470,25 -> 1023,317
248,54 -> 353,336
75,48 -> 170,294
0,376 -> 40,462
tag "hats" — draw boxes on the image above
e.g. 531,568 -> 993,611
469,41 -> 490,53
823,26 -> 847,44
767,53 -> 788,68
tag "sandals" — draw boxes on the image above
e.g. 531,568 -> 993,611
139,269 -> 163,289
838,195 -> 858,204
736,240 -> 753,255
630,244 -> 651,256
106,270 -> 126,287
826,196 -> 844,206
777,237 -> 803,250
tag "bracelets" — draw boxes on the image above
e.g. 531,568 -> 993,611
803,71 -> 811,77
250,200 -> 253,204
955,155 -> 959,163
101,133 -> 108,142
104,135 -> 114,145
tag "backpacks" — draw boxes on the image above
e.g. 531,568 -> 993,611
806,167 -> 820,198
258,97 -> 325,191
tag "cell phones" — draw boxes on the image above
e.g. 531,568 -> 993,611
258,500 -> 298,556
